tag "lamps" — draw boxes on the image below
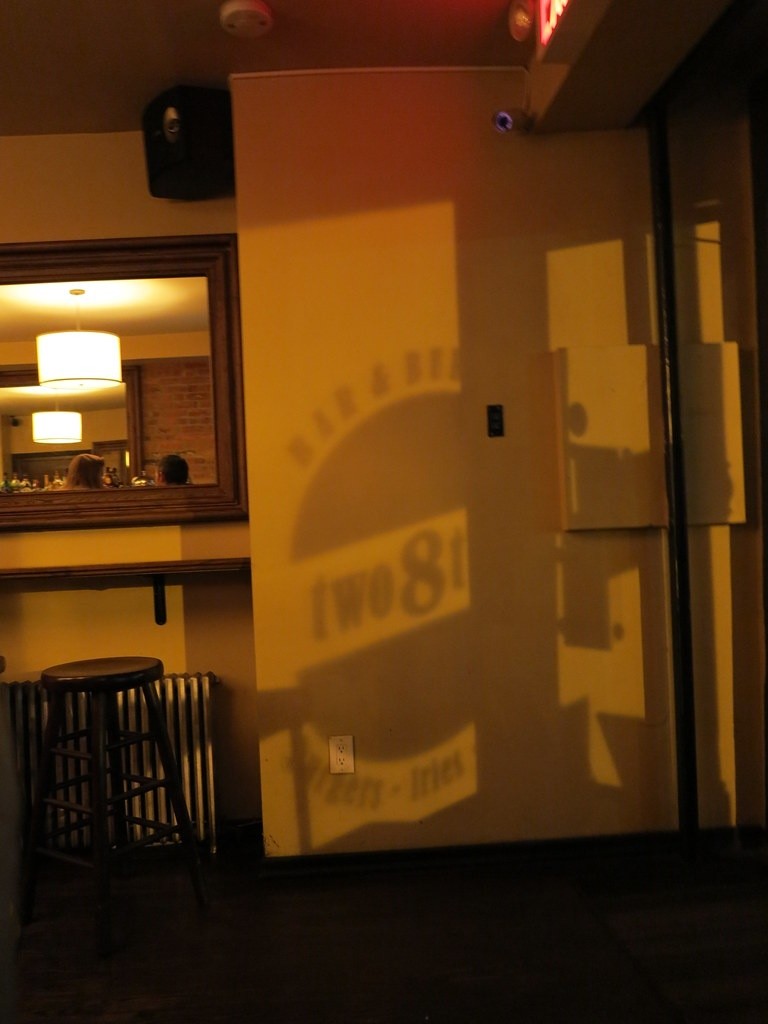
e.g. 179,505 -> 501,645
35,289 -> 122,390
31,391 -> 83,444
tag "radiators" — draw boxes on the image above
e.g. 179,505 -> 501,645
0,672 -> 222,855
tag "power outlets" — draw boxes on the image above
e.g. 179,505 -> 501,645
329,735 -> 355,774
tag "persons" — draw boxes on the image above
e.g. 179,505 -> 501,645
63,453 -> 106,492
155,454 -> 189,486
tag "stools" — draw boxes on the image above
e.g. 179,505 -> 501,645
23,657 -> 210,964
